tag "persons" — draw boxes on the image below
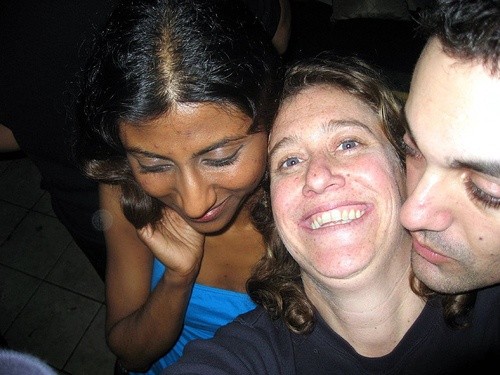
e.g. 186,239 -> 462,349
157,56 -> 500,374
394,0 -> 500,294
62,1 -> 317,374
